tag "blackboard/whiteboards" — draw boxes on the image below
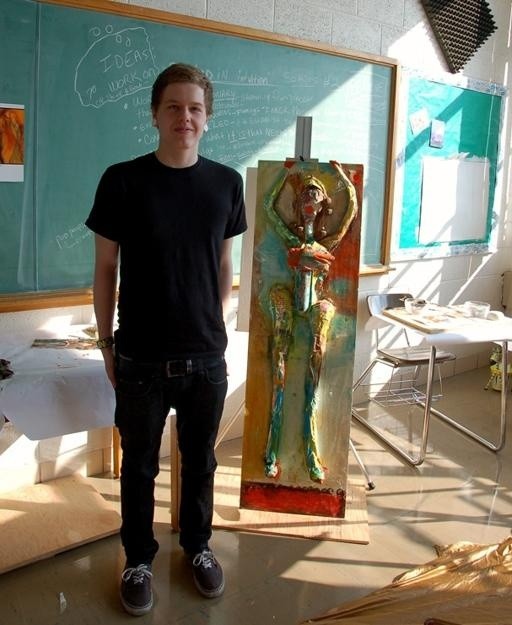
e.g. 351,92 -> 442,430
0,0 -> 399,313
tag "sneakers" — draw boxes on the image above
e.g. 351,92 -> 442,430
191,548 -> 225,597
120,564 -> 153,616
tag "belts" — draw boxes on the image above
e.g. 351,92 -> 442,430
167,356 -> 225,377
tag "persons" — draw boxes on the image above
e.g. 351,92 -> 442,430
84,61 -> 248,617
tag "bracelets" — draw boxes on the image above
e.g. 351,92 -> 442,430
97,337 -> 114,349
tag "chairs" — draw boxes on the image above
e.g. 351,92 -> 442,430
362,293 -> 457,409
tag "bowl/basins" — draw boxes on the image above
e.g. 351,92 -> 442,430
405,298 -> 429,314
464,300 -> 490,322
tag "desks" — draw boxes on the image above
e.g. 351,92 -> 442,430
352,307 -> 512,465
0,327 -> 252,533
